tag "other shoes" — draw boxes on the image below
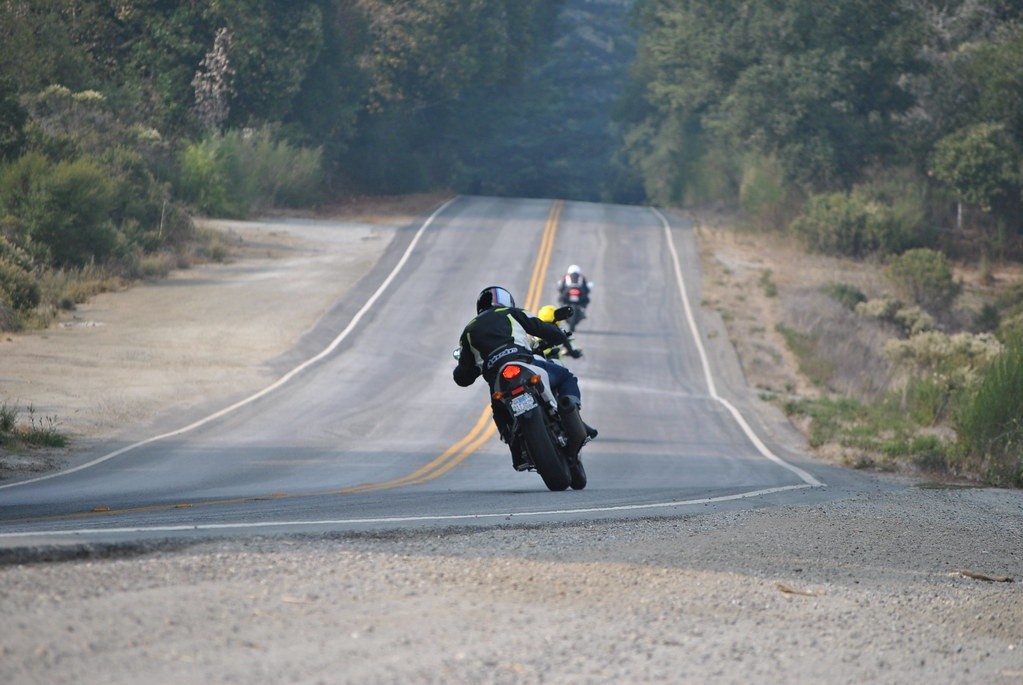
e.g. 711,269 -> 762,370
583,421 -> 598,439
508,434 -> 526,471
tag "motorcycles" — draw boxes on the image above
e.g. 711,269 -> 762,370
559,282 -> 593,331
452,306 -> 599,492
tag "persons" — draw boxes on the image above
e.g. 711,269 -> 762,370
453,286 -> 582,471
557,264 -> 590,308
535,305 -> 576,367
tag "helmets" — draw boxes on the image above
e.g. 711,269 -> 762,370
477,287 -> 515,315
568,264 -> 581,275
538,304 -> 560,325
